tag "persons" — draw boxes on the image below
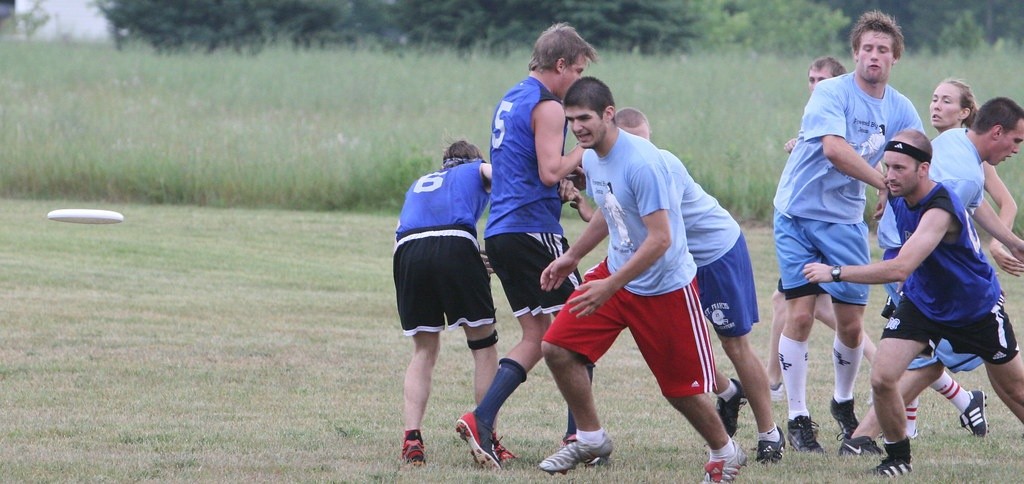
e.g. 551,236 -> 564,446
767,10 -> 1024,455
803,132 -> 1024,478
541,76 -> 748,484
558,108 -> 787,465
394,140 -> 518,465
456,23 -> 614,469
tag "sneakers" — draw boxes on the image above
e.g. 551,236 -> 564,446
769,382 -> 786,402
716,378 -> 741,438
959,391 -> 990,439
788,415 -> 826,455
829,397 -> 859,440
838,434 -> 886,457
401,429 -> 426,466
869,458 -> 913,478
562,434 -> 576,446
454,412 -> 502,472
755,426 -> 785,465
539,432 -> 614,476
470,433 -> 516,463
699,440 -> 747,484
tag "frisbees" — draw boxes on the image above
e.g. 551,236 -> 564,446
45,208 -> 125,225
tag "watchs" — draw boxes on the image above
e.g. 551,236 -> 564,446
830,264 -> 842,282
